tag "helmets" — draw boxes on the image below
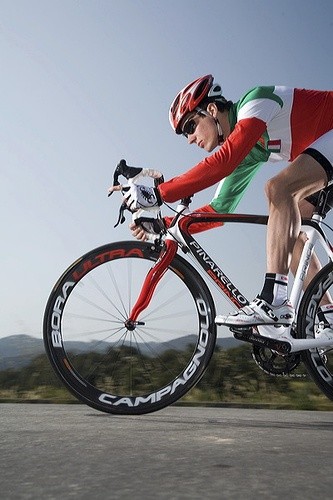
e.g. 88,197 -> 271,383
167,74 -> 222,135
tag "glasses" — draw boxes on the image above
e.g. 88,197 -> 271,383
181,101 -> 216,138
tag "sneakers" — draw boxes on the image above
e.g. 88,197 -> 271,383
214,294 -> 296,328
314,316 -> 333,356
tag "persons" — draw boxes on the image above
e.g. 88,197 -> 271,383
107,73 -> 333,359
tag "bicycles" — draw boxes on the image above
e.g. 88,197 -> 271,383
42,159 -> 333,415
